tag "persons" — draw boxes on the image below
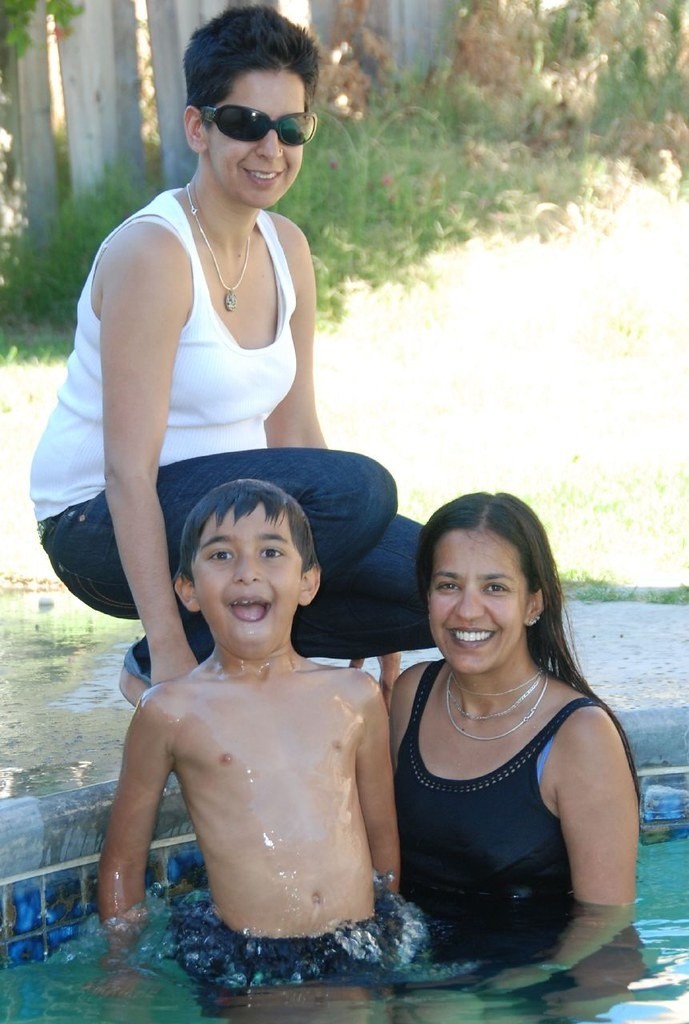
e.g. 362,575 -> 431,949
389,496 -> 638,904
98,474 -> 401,940
31,7 -> 438,707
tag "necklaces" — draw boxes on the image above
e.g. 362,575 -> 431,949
186,182 -> 250,311
446,668 -> 548,740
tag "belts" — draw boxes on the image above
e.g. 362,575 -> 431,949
37,515 -> 59,546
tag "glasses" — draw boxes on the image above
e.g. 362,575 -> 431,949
199,104 -> 318,146
